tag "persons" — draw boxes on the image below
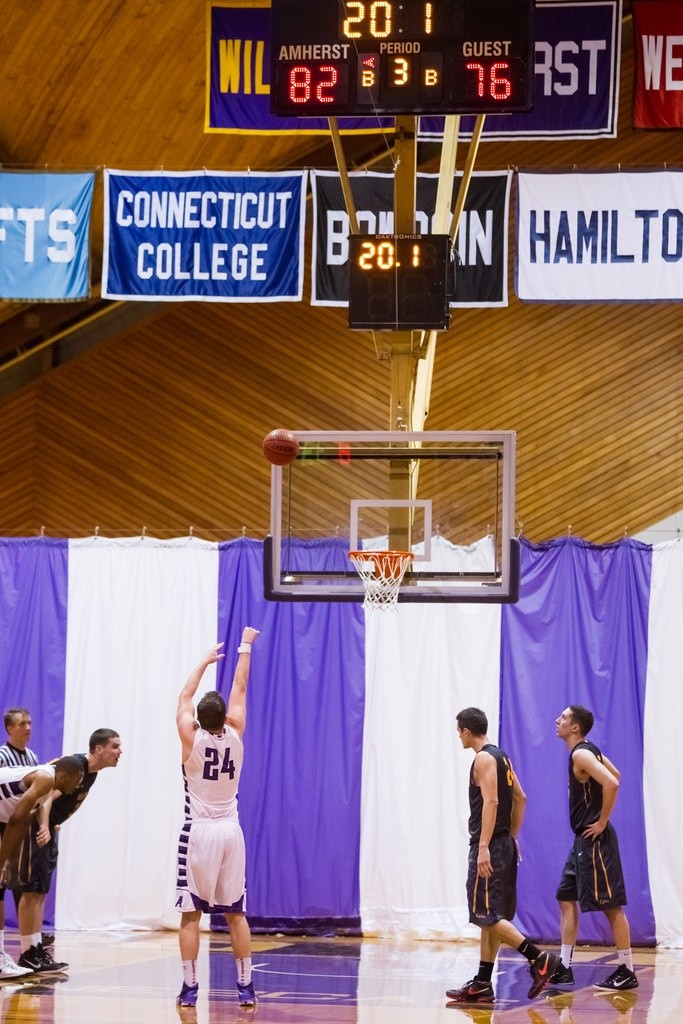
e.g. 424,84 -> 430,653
447,708 -> 562,1002
0,756 -> 85,977
545,704 -> 640,992
0,708 -> 55,951
178,625 -> 263,1008
10,729 -> 121,972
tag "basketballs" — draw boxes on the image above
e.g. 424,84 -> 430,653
261,429 -> 299,467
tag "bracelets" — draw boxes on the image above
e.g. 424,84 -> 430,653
238,642 -> 252,653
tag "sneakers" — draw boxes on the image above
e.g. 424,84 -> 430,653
0,953 -> 35,981
175,982 -> 199,1007
592,990 -> 640,1012
527,1008 -> 547,1024
539,986 -> 574,1009
542,957 -> 574,986
593,964 -> 640,992
527,950 -> 562,999
18,971 -> 69,987
445,999 -> 494,1024
235,979 -> 260,1006
445,976 -> 496,1001
17,943 -> 70,974
